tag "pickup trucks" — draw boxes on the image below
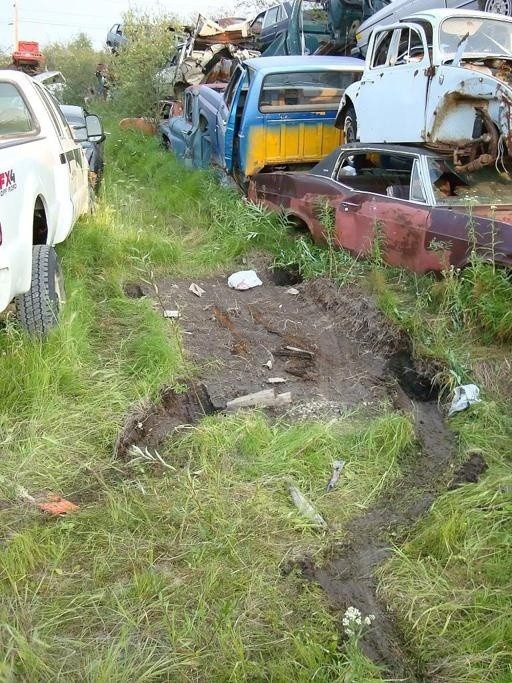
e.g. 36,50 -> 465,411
1,69 -> 105,341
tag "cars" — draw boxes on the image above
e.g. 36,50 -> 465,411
60,104 -> 105,194
233,141 -> 512,278
